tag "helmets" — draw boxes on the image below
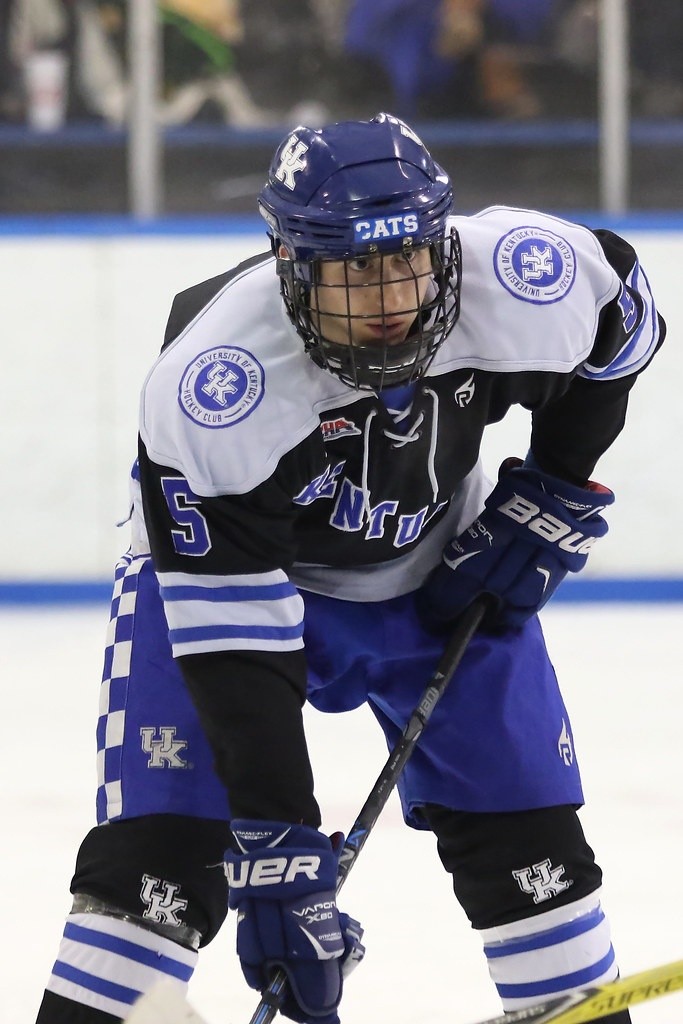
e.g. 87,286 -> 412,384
257,111 -> 463,394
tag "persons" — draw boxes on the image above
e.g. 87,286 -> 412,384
0,0 -> 585,189
35,111 -> 667,1024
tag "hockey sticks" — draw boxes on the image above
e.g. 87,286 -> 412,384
482,956 -> 680,1024
250,600 -> 488,1024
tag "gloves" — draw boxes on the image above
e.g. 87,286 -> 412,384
424,457 -> 615,628
223,817 -> 366,1024
